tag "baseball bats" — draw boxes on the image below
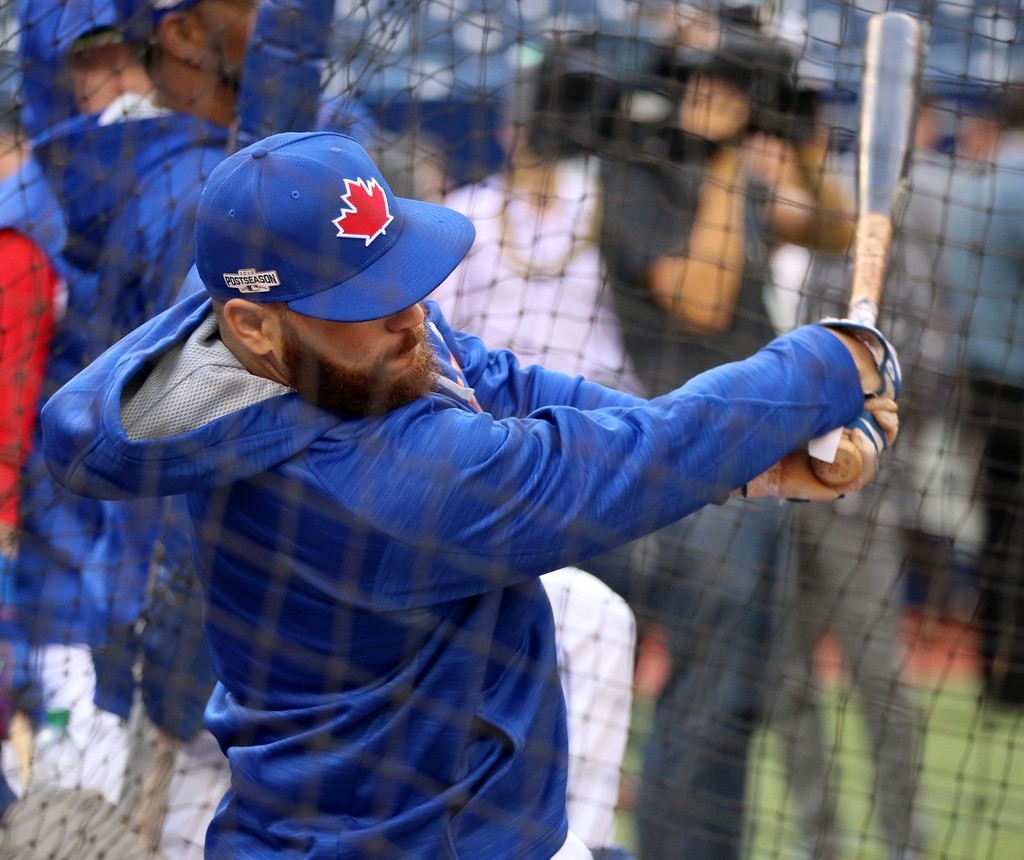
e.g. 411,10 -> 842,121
811,9 -> 922,489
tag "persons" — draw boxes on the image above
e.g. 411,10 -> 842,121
1,0 -> 1024,860
38,125 -> 902,860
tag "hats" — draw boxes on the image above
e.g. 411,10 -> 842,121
193,131 -> 476,323
